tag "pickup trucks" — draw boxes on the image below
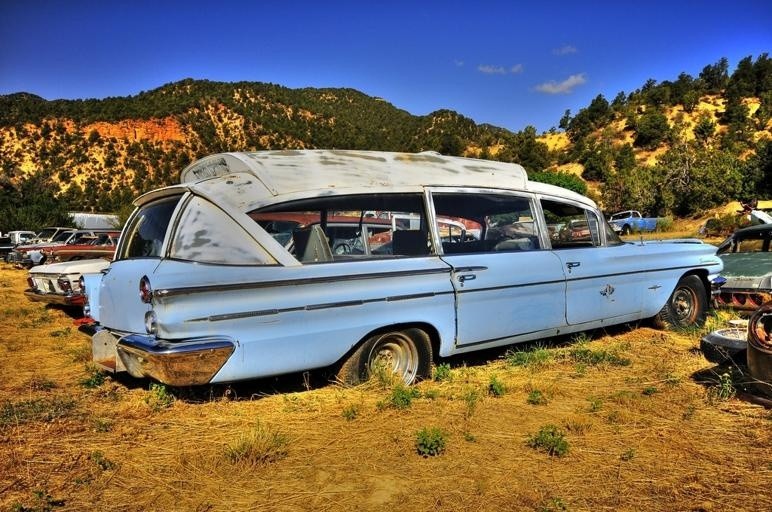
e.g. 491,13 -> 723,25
608,209 -> 657,238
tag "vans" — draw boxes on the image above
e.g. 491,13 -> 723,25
74,148 -> 726,390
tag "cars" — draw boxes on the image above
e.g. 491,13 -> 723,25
24,255 -> 113,306
557,218 -> 598,242
547,223 -> 568,239
513,221 -> 537,235
0,224 -> 120,266
247,213 -> 407,267
